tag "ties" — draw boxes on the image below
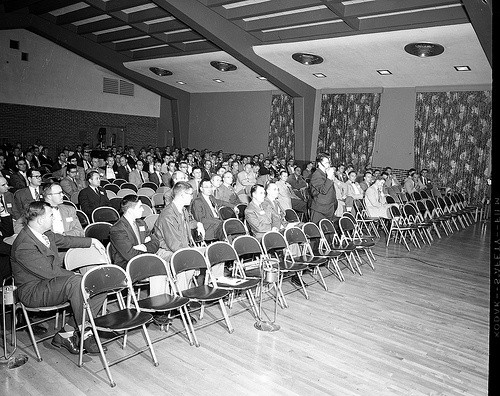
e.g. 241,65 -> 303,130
0,195 -> 6,211
180,209 -> 190,246
139,171 -> 144,183
133,219 -> 142,245
94,187 -> 99,194
367,180 -> 371,186
259,203 -> 264,211
86,160 -> 90,168
285,182 -> 291,193
34,188 -> 40,202
209,196 -> 216,209
42,234 -> 50,248
228,185 -> 237,197
72,178 -> 77,187
126,165 -> 129,173
378,189 -> 380,203
412,178 -> 415,187
273,199 -> 284,221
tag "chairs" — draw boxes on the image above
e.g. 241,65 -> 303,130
0,179 -> 478,387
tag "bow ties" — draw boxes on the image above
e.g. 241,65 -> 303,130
352,181 -> 355,183
51,206 -> 58,211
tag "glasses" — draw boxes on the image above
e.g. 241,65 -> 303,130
18,162 -> 26,166
31,175 -> 42,179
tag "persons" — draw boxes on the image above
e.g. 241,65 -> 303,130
486,176 -> 491,205
0,143 -> 433,354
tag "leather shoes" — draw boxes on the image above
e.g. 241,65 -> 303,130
51,333 -> 86,354
16,318 -> 47,335
73,330 -> 107,353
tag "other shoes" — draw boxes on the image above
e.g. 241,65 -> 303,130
176,309 -> 197,325
152,310 -> 171,326
291,275 -> 308,286
